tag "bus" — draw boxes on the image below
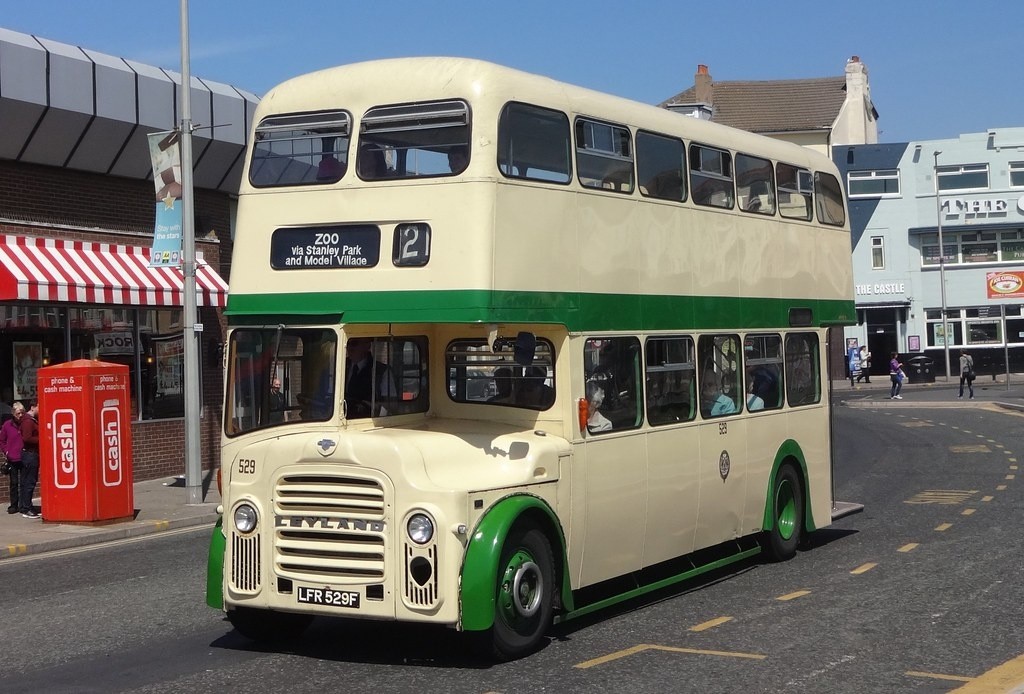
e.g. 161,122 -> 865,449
211,55 -> 860,653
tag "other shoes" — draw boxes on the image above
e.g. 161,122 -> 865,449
5,505 -> 20,514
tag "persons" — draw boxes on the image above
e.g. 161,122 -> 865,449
486,366 -> 556,411
0,400 -> 42,519
584,359 -> 804,435
855,345 -> 872,384
346,337 -> 396,419
269,379 -> 288,425
956,349 -> 975,400
889,353 -> 904,399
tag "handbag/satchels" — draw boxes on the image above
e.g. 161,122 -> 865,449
0,452 -> 11,475
968,369 -> 977,380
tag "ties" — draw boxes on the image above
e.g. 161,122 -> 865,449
348,363 -> 359,390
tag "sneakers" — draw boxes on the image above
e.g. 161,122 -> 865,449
20,507 -> 41,520
27,504 -> 43,516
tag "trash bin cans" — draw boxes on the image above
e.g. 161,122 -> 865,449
908,355 -> 936,383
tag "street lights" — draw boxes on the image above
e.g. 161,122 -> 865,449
934,150 -> 952,383
